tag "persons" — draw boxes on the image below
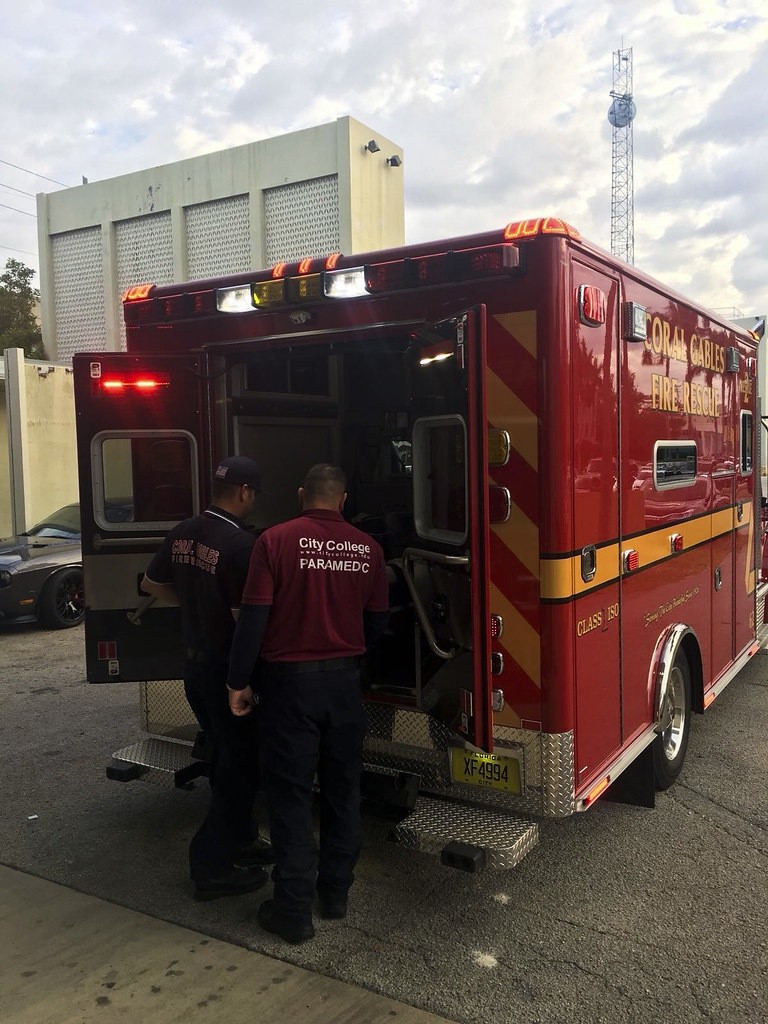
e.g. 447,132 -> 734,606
141,457 -> 278,903
225,465 -> 391,944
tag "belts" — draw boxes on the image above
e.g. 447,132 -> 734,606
272,656 -> 365,675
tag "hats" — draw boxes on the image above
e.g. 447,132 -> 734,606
214,454 -> 273,493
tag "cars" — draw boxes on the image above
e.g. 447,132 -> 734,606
0,496 -> 133,632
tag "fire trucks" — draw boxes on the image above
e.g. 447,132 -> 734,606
69,215 -> 768,875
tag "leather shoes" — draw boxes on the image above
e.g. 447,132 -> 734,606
233,834 -> 277,865
193,865 -> 270,900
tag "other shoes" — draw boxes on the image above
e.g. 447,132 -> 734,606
258,898 -> 315,944
319,895 -> 348,920
